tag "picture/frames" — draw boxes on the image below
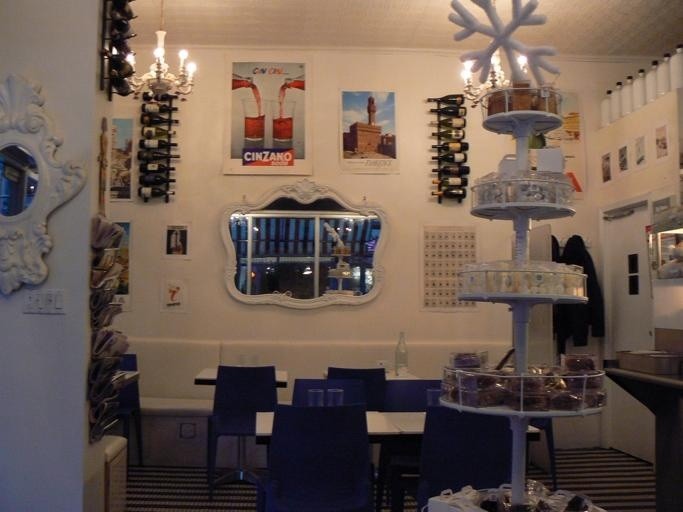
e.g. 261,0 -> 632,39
222,52 -> 313,176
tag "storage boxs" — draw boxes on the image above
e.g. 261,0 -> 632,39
618,327 -> 683,375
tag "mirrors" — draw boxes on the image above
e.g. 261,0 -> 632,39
220,178 -> 390,309
0,73 -> 86,295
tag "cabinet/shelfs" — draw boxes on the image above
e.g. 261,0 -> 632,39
437,87 -> 608,512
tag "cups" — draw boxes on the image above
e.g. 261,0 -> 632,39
242,98 -> 267,142
271,100 -> 296,143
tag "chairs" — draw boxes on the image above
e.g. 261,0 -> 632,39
110,354 -> 144,467
207,366 -> 513,512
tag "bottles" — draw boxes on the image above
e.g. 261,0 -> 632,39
282,75 -> 305,90
232,74 -> 252,91
598,43 -> 682,128
99,0 -> 133,96
395,331 -> 409,377
427,94 -> 470,199
136,91 -> 180,200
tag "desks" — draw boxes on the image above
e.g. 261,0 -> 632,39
604,367 -> 683,512
109,370 -> 140,389
195,369 -> 287,484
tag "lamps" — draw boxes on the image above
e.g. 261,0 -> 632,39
125,0 -> 197,95
459,0 -> 528,109
